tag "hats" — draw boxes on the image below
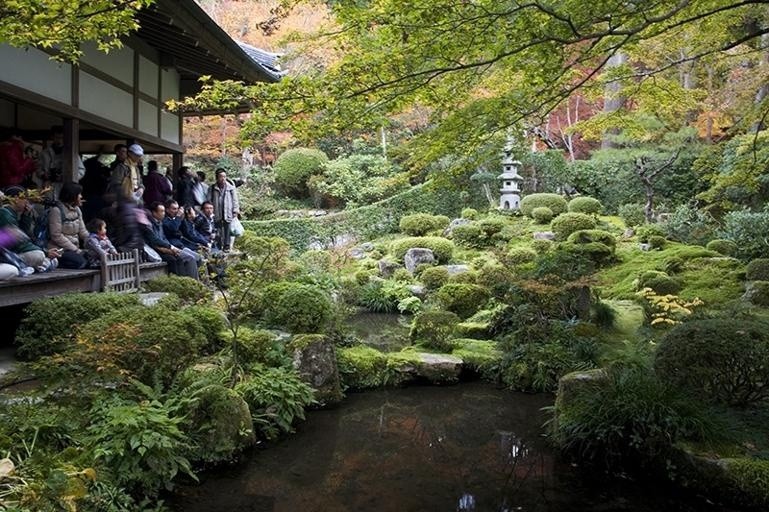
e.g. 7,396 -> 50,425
128,144 -> 145,157
2,186 -> 34,204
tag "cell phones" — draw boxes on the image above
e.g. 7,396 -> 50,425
57,248 -> 65,255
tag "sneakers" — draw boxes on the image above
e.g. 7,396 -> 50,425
209,271 -> 229,280
217,279 -> 230,289
19,257 -> 59,277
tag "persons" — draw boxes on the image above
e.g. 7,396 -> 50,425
2,120 -> 240,285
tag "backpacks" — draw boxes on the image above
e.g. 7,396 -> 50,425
32,206 -> 81,248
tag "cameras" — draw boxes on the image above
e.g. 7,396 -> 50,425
182,204 -> 191,216
